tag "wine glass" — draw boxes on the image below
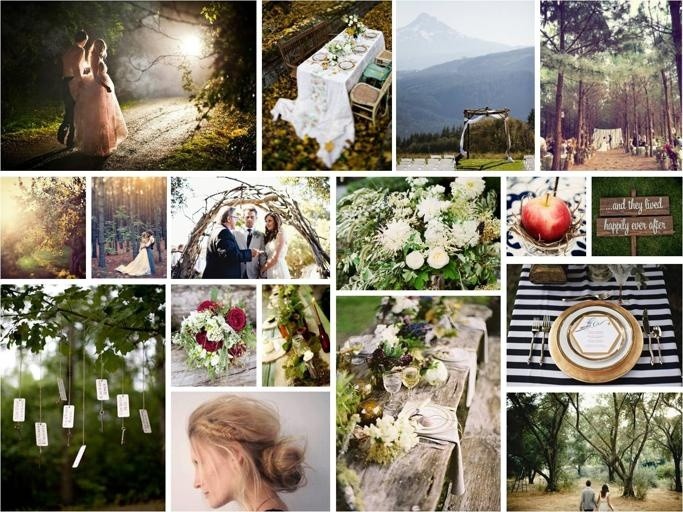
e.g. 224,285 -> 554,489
607,264 -> 637,307
382,362 -> 449,410
348,336 -> 365,366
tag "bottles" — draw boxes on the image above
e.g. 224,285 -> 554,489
348,15 -> 358,39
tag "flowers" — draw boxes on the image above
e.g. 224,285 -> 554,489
340,14 -> 366,38
171,287 -> 255,384
325,41 -> 344,62
336,410 -> 419,466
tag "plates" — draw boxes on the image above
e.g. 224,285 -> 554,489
547,300 -> 644,387
313,32 -> 377,70
411,404 -> 453,435
434,347 -> 471,362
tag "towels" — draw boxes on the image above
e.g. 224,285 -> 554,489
394,405 -> 465,495
453,348 -> 477,407
470,317 -> 488,363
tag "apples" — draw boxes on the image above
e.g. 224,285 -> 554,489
521,193 -> 570,241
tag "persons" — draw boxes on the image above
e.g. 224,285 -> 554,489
579,480 -> 615,512
201,207 -> 290,279
114,228 -> 156,278
57,28 -> 127,157
187,392 -> 305,512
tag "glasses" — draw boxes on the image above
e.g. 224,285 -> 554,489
229,214 -> 237,217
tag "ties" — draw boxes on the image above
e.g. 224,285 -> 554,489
247,229 -> 252,248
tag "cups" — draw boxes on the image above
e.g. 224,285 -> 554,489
587,265 -> 612,283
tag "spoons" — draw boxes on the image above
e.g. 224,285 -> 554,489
652,324 -> 664,365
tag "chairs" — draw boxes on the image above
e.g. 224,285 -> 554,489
360,64 -> 391,89
349,71 -> 391,127
375,50 -> 391,67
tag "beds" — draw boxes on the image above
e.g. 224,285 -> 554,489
336,305 -> 492,511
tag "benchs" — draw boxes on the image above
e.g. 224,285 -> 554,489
278,22 -> 338,88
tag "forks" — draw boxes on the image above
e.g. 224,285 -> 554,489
559,290 -> 614,300
527,312 -> 551,368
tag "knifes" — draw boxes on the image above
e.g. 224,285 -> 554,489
643,307 -> 655,368
